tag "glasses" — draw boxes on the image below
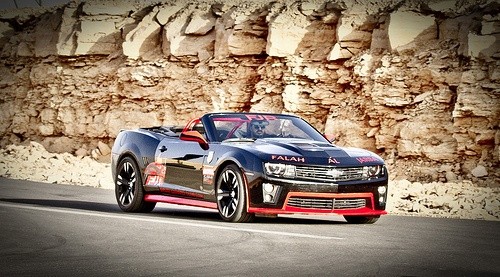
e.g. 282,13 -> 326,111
254,125 -> 266,128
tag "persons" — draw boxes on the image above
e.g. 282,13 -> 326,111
247,120 -> 290,138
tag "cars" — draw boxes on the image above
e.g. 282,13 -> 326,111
110,113 -> 388,222
